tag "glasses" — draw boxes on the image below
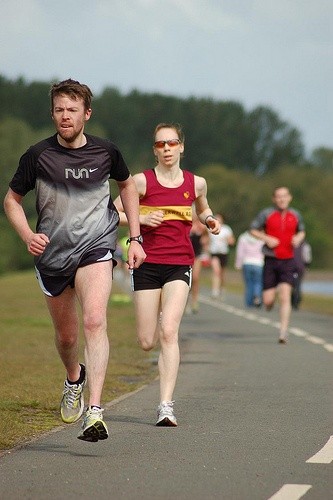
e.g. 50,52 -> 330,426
155,139 -> 183,147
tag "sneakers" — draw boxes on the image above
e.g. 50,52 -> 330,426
60,362 -> 87,423
76,406 -> 109,443
156,399 -> 177,427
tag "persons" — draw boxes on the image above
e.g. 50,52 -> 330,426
119,231 -> 131,293
235,223 -> 266,308
184,205 -> 211,314
3,78 -> 147,443
209,213 -> 235,297
113,122 -> 221,426
248,184 -> 306,344
292,242 -> 312,311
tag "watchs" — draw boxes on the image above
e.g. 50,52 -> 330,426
205,215 -> 217,229
126,234 -> 144,245
